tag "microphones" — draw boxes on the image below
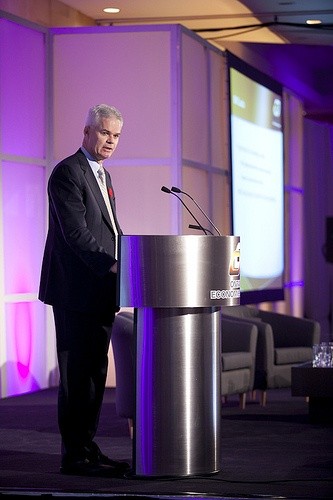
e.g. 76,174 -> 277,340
171,186 -> 221,235
189,224 -> 214,235
161,186 -> 207,235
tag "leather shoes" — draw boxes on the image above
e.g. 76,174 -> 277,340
62,455 -> 130,479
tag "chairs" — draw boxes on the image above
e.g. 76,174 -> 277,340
111,311 -> 258,439
221,306 -> 321,407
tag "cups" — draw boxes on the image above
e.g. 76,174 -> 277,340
312,342 -> 333,368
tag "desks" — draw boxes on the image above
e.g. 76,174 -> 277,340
291,362 -> 333,424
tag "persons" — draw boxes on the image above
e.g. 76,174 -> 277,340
39,103 -> 131,476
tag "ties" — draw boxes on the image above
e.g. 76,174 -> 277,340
98,169 -> 118,235
